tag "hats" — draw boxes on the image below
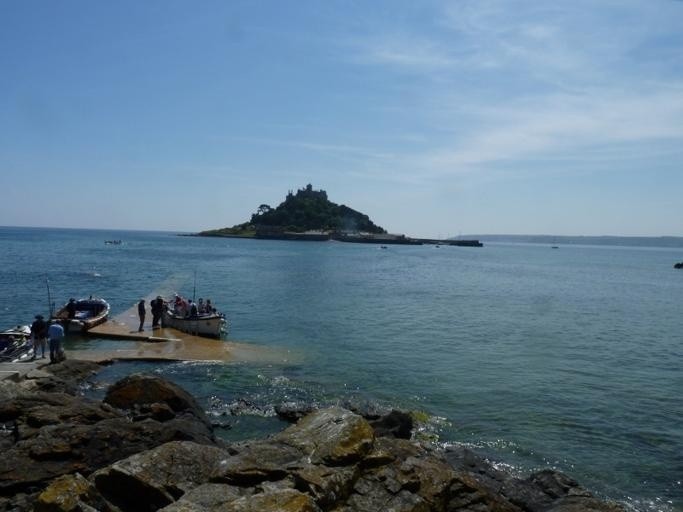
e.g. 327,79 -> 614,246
69,298 -> 76,301
35,314 -> 45,319
157,295 -> 164,300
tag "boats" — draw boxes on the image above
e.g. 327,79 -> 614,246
162,309 -> 223,338
49,297 -> 110,332
1,325 -> 35,362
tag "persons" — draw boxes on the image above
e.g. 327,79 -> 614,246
45,319 -> 65,364
64,296 -> 78,319
136,297 -> 146,332
26,313 -> 49,361
149,293 -> 216,330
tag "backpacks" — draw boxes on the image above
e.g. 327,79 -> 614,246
191,303 -> 198,315
